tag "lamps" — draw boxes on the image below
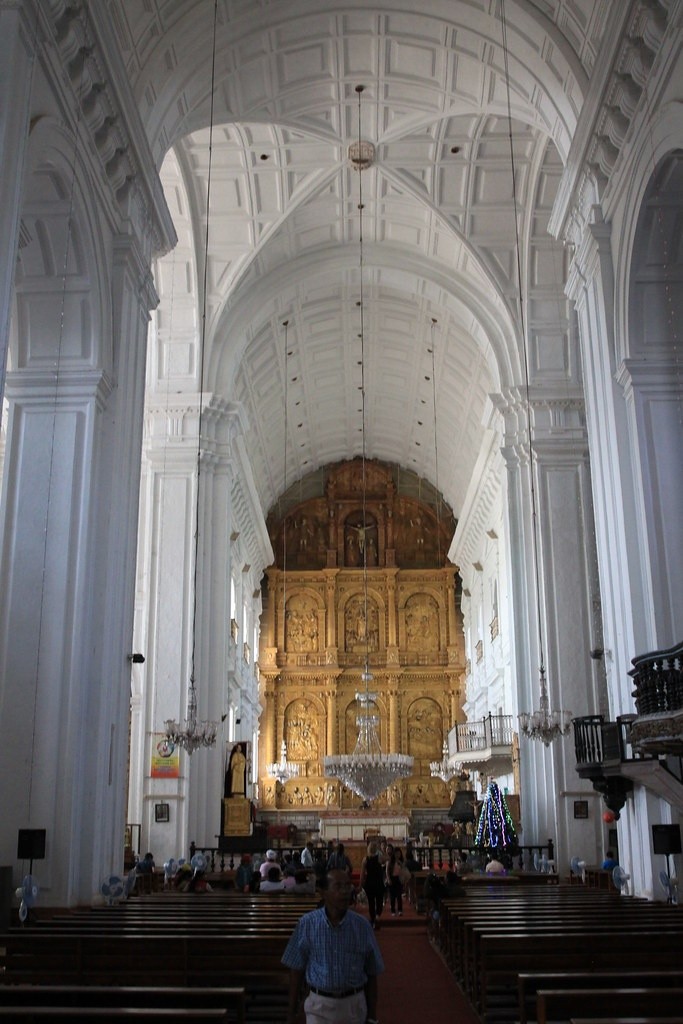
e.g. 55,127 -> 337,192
498,0 -> 572,747
164,0 -> 221,756
324,84 -> 415,805
269,317 -> 300,789
431,322 -> 464,786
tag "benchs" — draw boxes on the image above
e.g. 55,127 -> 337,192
411,870 -> 683,1024
0,870 -> 320,1024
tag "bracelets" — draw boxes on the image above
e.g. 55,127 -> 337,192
367,1018 -> 377,1024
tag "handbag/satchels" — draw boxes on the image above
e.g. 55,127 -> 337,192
384,878 -> 391,887
357,889 -> 366,904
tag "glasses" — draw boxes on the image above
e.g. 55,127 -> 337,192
325,884 -> 352,894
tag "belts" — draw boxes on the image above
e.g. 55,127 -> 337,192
311,986 -> 363,999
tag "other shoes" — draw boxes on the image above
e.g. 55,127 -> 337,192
371,923 -> 375,928
375,915 -> 380,922
399,911 -> 402,916
383,903 -> 385,908
392,913 -> 395,916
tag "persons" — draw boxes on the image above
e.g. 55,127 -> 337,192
274,870 -> 386,1024
124,820 -> 618,931
232,744 -> 252,794
263,518 -> 474,806
477,772 -> 488,794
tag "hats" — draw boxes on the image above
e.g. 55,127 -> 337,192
243,854 -> 251,861
399,867 -> 411,883
266,850 -> 276,859
